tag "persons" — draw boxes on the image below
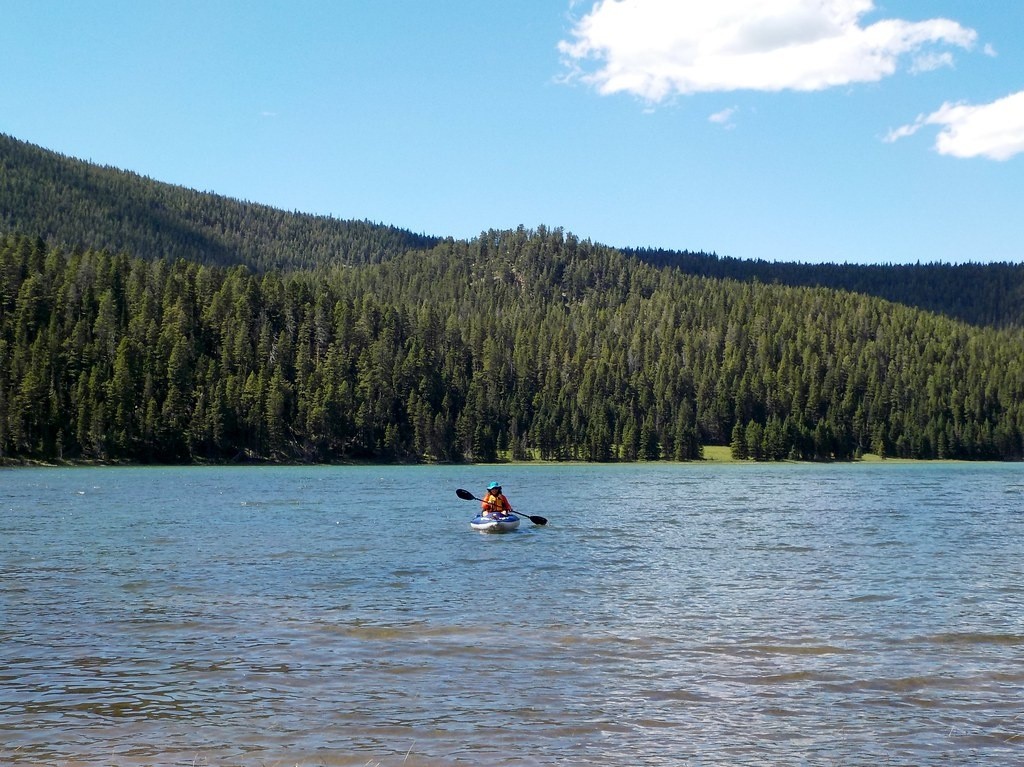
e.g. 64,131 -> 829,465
482,481 -> 512,517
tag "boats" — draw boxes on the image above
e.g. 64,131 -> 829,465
470,512 -> 520,534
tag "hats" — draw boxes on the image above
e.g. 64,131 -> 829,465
486,481 -> 502,491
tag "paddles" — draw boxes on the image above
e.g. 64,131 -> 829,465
455,489 -> 548,525
